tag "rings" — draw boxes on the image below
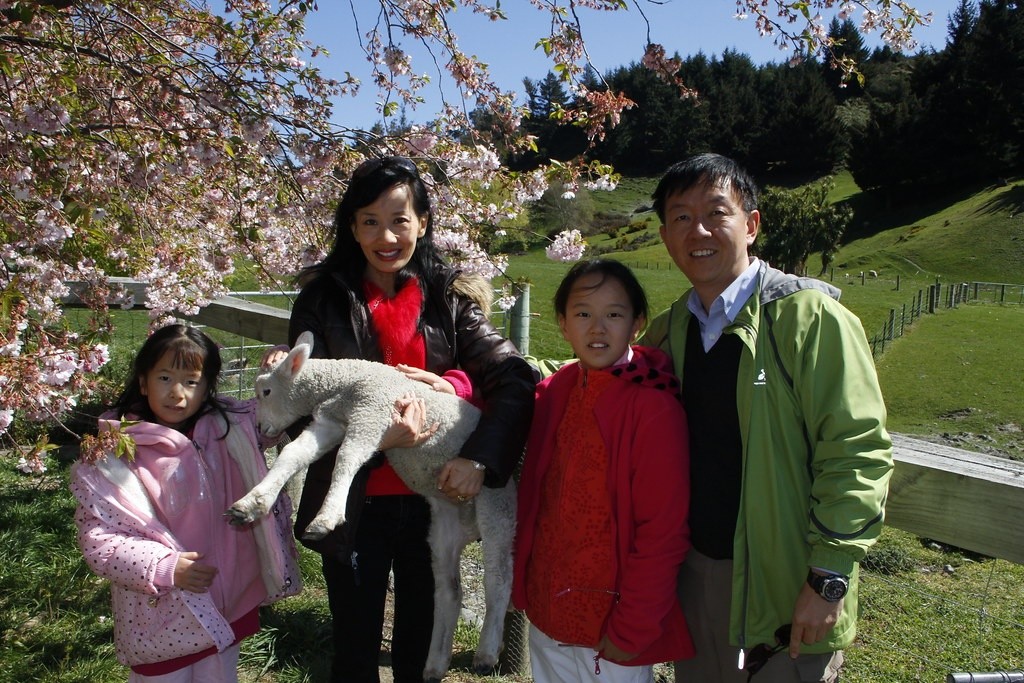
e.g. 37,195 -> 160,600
456,495 -> 466,504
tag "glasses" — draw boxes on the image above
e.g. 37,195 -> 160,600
352,155 -> 425,195
745,624 -> 793,683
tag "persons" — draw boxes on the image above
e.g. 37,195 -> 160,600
289,157 -> 536,683
392,254 -> 697,683
67,322 -> 305,683
521,153 -> 897,683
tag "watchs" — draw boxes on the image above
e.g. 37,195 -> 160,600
807,568 -> 851,602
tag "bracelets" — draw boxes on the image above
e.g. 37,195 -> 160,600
472,460 -> 487,471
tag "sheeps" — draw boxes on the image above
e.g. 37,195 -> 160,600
220,330 -> 521,681
